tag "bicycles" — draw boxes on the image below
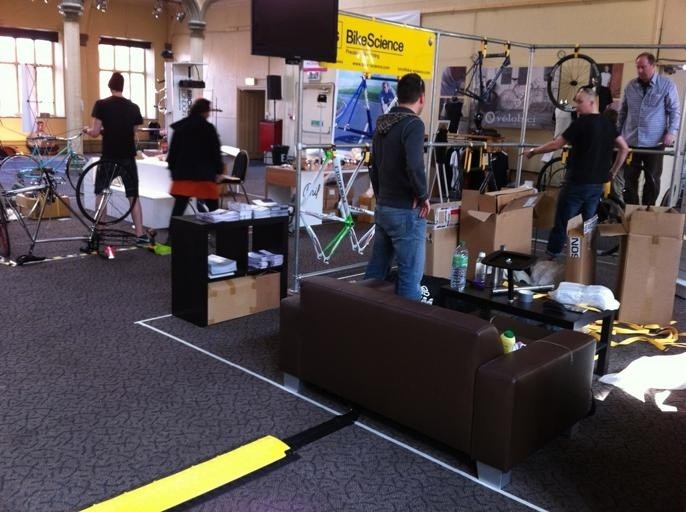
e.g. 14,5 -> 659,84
0,132 -> 139,267
497,77 -> 549,114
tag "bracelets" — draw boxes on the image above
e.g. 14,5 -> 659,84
82,126 -> 89,134
529,147 -> 534,156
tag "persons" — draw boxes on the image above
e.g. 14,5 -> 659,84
515,86 -> 631,264
603,106 -> 626,205
360,72 -> 432,305
76,70 -> 157,248
165,96 -> 224,243
25,119 -> 59,156
616,51 -> 682,206
379,79 -> 396,115
598,64 -> 611,89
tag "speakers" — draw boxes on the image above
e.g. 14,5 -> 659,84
266,74 -> 283,100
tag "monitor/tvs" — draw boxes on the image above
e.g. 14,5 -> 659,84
250,0 -> 339,65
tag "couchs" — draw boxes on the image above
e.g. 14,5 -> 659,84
279,276 -> 596,482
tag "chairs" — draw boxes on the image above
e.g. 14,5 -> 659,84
214,149 -> 249,209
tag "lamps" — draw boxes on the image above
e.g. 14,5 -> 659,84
175,8 -> 185,23
152,0 -> 163,25
95,0 -> 107,13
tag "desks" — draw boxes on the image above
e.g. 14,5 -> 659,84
264,165 -> 297,200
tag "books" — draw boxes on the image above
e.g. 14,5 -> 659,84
207,251 -> 239,279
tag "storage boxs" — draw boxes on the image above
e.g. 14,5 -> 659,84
15,192 -> 72,221
323,186 -> 376,224
596,204 -> 685,329
424,183 -> 563,280
565,214 -> 599,285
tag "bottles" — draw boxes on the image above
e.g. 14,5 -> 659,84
450,240 -> 469,291
500,329 -> 516,354
471,251 -> 488,289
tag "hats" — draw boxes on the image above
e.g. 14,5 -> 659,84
108,72 -> 125,92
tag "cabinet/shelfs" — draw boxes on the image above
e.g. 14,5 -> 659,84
170,204 -> 289,327
